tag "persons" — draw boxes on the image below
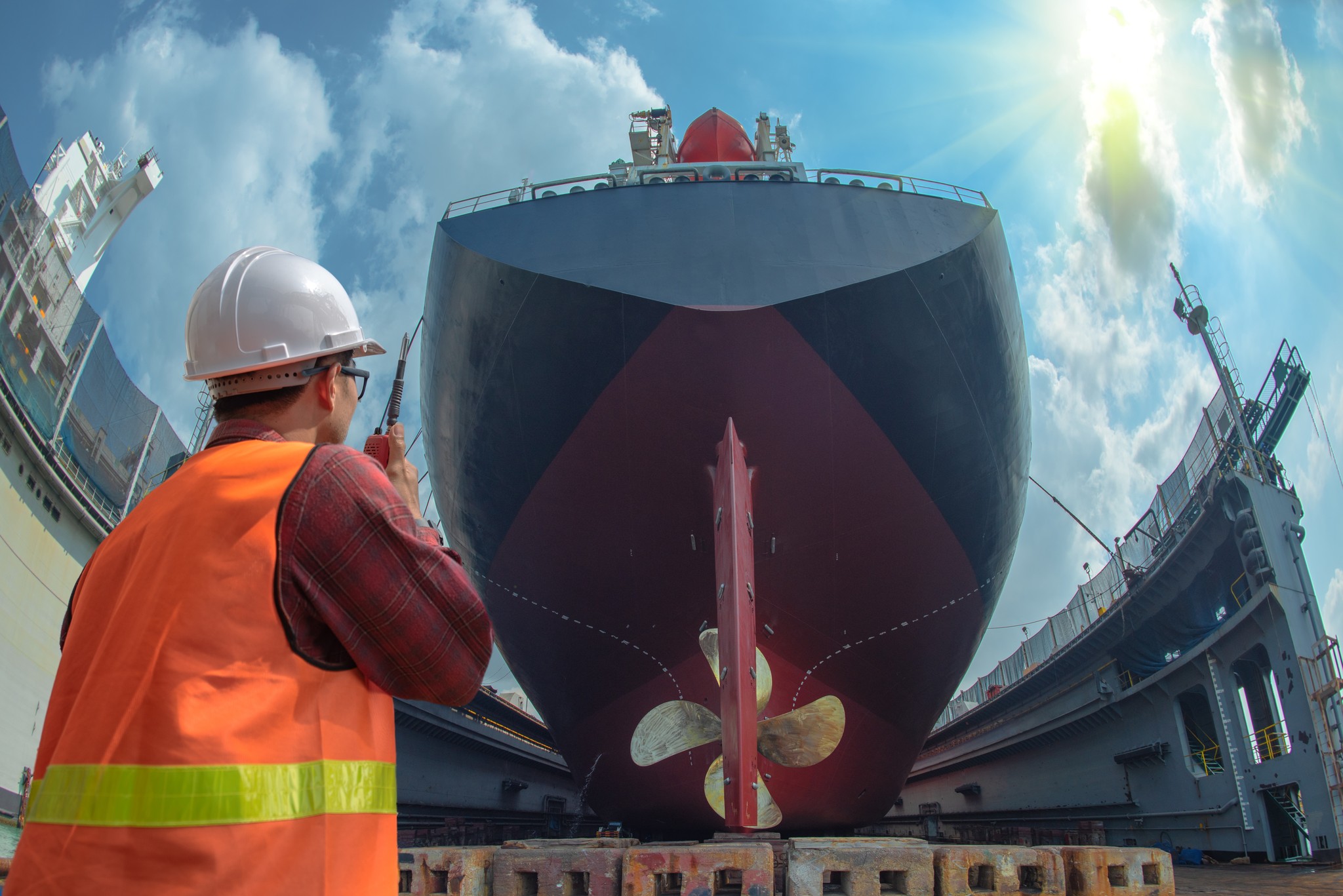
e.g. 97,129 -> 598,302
8,243 -> 496,896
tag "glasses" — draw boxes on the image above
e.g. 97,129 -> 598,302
301,365 -> 370,400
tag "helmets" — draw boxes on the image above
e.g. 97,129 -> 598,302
182,246 -> 387,382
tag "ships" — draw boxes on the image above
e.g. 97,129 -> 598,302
412,104 -> 1031,844
